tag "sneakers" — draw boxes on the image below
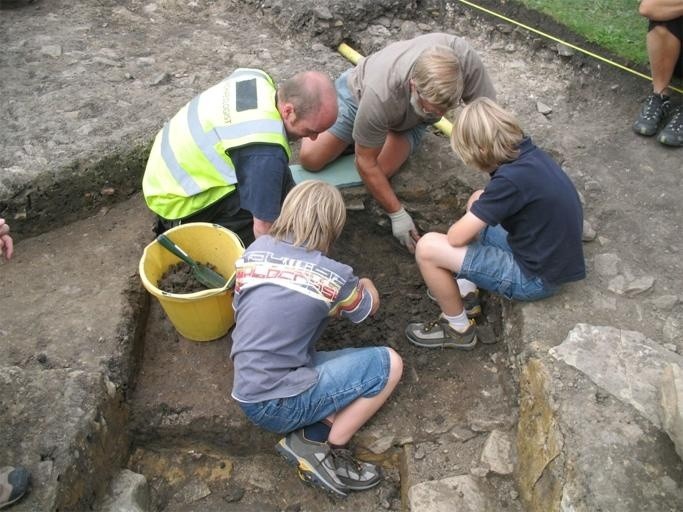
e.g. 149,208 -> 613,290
405,318 -> 477,351
276,426 -> 350,498
1,463 -> 33,508
297,450 -> 381,489
632,93 -> 669,136
364,190 -> 392,232
657,108 -> 683,147
429,287 -> 481,317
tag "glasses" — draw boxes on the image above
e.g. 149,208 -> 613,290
419,100 -> 461,114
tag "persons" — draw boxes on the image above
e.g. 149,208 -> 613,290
299,32 -> 499,256
0,218 -> 29,509
229,180 -> 404,498
405,96 -> 586,351
142,66 -> 339,240
632,0 -> 683,147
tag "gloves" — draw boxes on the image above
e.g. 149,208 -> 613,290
383,208 -> 420,255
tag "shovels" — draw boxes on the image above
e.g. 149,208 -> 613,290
159,235 -> 227,289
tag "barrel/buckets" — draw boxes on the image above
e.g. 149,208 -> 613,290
138,222 -> 246,342
